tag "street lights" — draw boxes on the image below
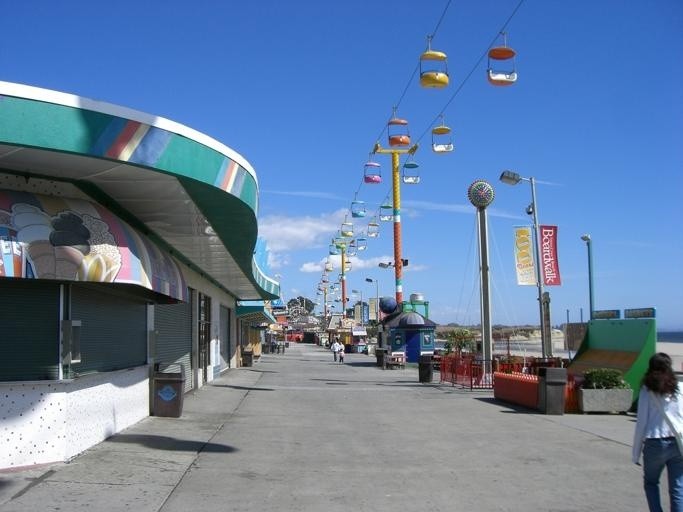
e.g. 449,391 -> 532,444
378,262 -> 402,308
366,276 -> 379,323
499,169 -> 550,360
581,233 -> 594,320
319,311 -> 327,321
333,296 -> 346,319
352,289 -> 364,328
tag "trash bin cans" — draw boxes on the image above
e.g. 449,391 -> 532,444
153,361 -> 186,418
376,348 -> 388,367
242,347 -> 254,367
419,354 -> 434,383
285,343 -> 289,348
538,367 -> 566,415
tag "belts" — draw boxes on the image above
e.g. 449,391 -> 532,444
648,435 -> 676,442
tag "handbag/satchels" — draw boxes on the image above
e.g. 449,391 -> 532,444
676,429 -> 683,461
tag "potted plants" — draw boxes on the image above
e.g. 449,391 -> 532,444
577,367 -> 633,414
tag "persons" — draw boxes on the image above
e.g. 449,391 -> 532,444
339,341 -> 345,352
331,339 -> 340,361
339,349 -> 345,363
631,352 -> 683,512
